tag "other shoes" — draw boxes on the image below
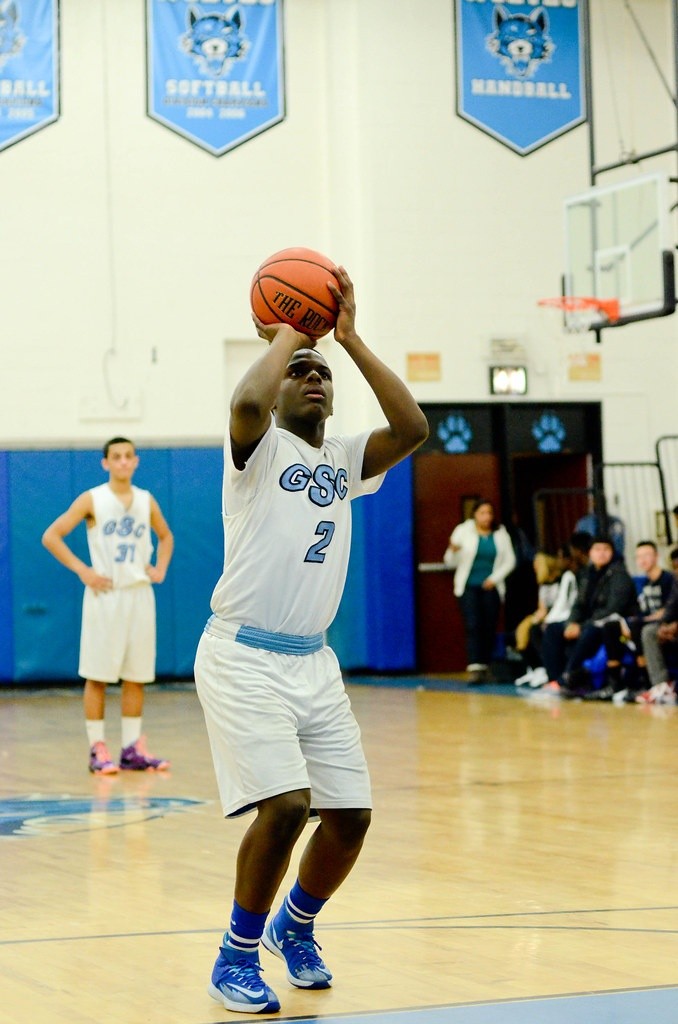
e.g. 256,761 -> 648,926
514,666 -> 534,686
466,669 -> 484,683
529,667 -> 548,687
485,668 -> 500,683
531,681 -> 677,704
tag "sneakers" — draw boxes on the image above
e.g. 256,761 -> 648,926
206,932 -> 281,1014
88,742 -> 120,774
261,917 -> 333,989
118,735 -> 171,772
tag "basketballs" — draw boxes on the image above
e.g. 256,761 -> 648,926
249,245 -> 344,342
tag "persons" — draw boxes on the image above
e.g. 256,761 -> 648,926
443,500 -> 517,673
514,530 -> 678,702
193,265 -> 429,1013
41,437 -> 174,775
575,492 -> 624,554
